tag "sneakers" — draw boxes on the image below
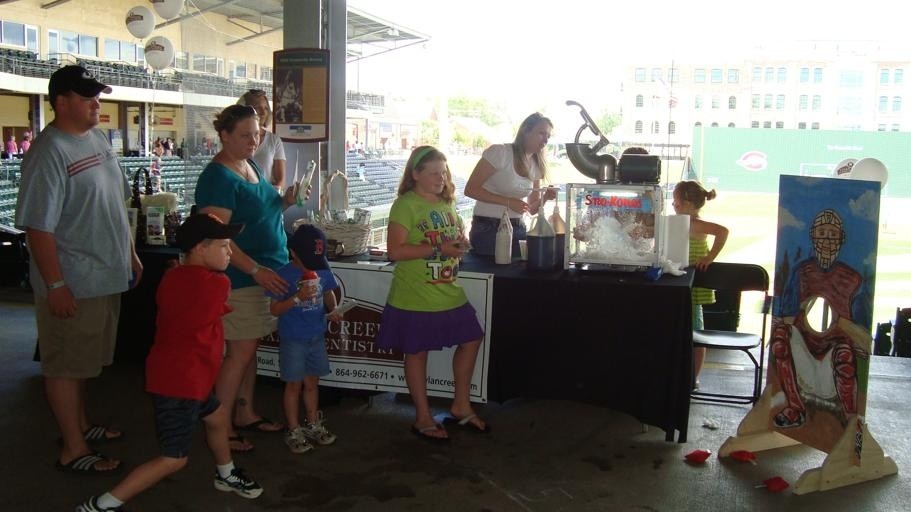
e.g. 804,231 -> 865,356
841,415 -> 864,459
74,496 -> 118,512
772,410 -> 810,431
301,410 -> 339,446
211,464 -> 264,499
284,427 -> 313,454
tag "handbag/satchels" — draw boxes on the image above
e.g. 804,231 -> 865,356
131,165 -> 182,248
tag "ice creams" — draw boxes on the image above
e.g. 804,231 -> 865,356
301,272 -> 320,304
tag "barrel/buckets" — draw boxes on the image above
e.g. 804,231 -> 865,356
547,205 -> 566,270
525,206 -> 556,272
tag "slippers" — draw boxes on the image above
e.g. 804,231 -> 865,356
58,453 -> 124,477
228,433 -> 258,457
410,424 -> 452,443
84,423 -> 124,445
233,415 -> 282,434
442,413 -> 491,436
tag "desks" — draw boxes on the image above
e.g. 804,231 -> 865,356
134,230 -> 694,444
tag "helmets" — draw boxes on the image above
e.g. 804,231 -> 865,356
809,209 -> 847,269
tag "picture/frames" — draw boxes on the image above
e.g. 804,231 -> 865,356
272,48 -> 331,144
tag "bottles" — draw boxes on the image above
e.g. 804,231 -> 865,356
497,208 -> 513,233
495,219 -> 512,264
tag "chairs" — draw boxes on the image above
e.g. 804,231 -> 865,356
691,261 -> 770,409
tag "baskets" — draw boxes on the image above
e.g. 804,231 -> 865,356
291,168 -> 373,257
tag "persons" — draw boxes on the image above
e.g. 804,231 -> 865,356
75,213 -> 263,512
374,146 -> 494,442
345,138 -> 373,187
14,66 -> 144,477
195,105 -> 312,456
4,128 -> 33,160
266,224 -> 338,455
145,132 -> 218,190
670,180 -> 729,389
771,210 -> 868,457
464,112 -> 557,255
236,89 -> 287,190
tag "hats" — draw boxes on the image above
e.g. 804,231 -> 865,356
286,224 -> 331,272
173,211 -> 245,255
47,65 -> 112,100
